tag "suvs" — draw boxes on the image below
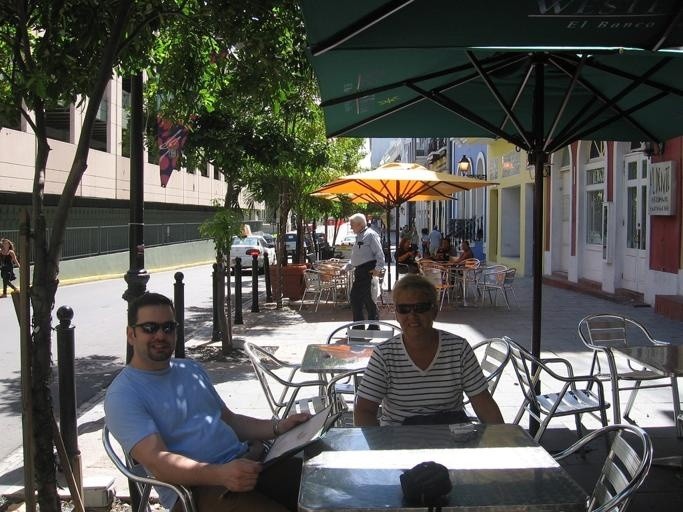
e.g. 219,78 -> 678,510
281,232 -> 311,254
340,237 -> 356,246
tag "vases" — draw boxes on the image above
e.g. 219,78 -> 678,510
270,264 -> 307,300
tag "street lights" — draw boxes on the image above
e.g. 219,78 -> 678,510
458,155 -> 471,254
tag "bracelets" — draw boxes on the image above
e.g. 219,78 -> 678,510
273,420 -> 280,437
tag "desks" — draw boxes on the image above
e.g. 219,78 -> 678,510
295,424 -> 590,510
449,268 -> 480,307
301,342 -> 376,412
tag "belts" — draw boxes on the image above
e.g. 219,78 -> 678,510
354,259 -> 376,270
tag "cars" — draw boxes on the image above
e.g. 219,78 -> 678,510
223,236 -> 276,271
264,236 -> 276,248
314,233 -> 325,242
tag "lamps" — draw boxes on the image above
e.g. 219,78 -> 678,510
458,155 -> 469,175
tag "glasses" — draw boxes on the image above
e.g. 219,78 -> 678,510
129,321 -> 179,333
393,301 -> 436,314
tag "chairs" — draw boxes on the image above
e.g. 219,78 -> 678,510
480,267 -> 519,311
550,424 -> 654,512
324,367 -> 382,428
420,263 -> 452,311
326,321 -> 403,406
330,258 -> 351,288
243,341 -> 327,418
417,258 -> 446,283
579,312 -> 682,438
101,421 -> 199,512
452,258 -> 481,300
314,264 -> 349,303
503,334 -> 611,454
478,264 -> 507,305
298,269 -> 335,313
392,252 -> 410,277
463,336 -> 511,422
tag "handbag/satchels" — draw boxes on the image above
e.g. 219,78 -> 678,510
370,274 -> 381,300
402,411 -> 470,426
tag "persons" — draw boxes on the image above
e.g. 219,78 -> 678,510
103,293 -> 304,512
435,238 -> 457,261
339,212 -> 385,335
0,239 -> 20,298
394,238 -> 420,274
354,275 -> 505,428
427,226 -> 442,257
421,229 -> 429,258
448,242 -> 474,291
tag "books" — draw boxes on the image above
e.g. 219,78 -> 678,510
259,403 -> 343,468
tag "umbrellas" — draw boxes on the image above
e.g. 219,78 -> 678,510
298,1 -> 683,442
309,162 -> 500,287
309,193 -> 459,292
